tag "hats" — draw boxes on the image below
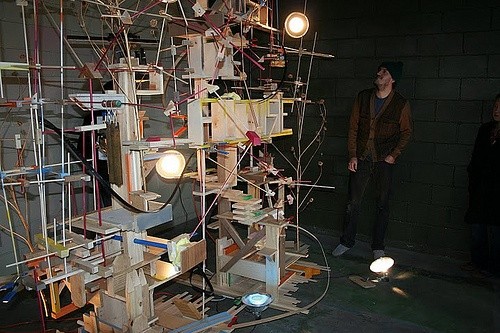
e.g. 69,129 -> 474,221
378,61 -> 403,83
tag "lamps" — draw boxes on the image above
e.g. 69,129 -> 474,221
241,292 -> 274,320
156,150 -> 185,180
284,11 -> 309,38
368,256 -> 394,282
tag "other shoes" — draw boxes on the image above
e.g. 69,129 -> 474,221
373,250 -> 385,260
332,244 -> 350,257
469,269 -> 496,279
464,263 -> 472,270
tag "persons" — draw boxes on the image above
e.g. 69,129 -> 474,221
460,93 -> 500,279
331,60 -> 410,260
